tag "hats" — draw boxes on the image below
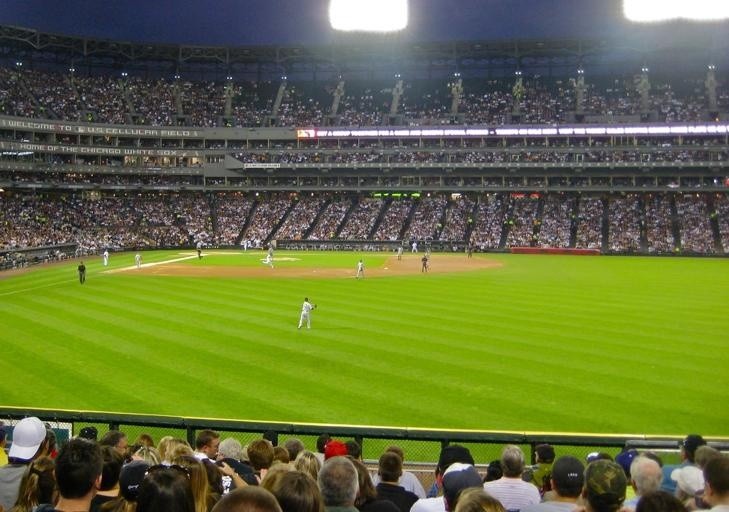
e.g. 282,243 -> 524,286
323,440 -> 349,459
584,459 -> 627,503
669,466 -> 707,498
552,455 -> 585,486
677,434 -> 708,452
7,417 -> 47,461
617,448 -> 639,475
119,459 -> 150,499
442,462 -> 482,500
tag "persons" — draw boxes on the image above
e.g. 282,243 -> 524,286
297,297 -> 316,329
0,412 -> 729,511
77,260 -> 86,284
0,61 -> 729,271
355,259 -> 366,279
420,254 -> 428,273
